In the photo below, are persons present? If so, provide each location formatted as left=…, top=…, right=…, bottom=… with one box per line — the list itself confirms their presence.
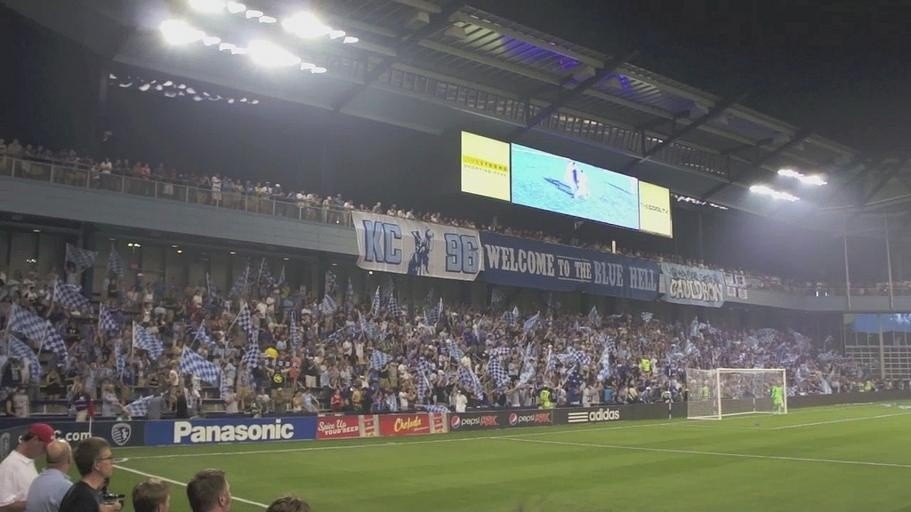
left=2, top=137, right=909, bottom=425
left=0, top=423, right=317, bottom=511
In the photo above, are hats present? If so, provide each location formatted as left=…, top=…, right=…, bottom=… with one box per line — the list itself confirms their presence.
left=30, top=423, right=55, bottom=448
